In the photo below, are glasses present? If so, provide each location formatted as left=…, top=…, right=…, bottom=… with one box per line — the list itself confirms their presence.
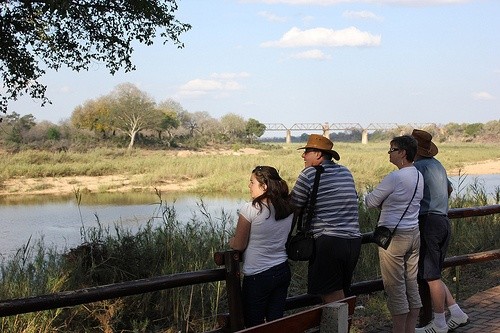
left=390, top=147, right=400, bottom=151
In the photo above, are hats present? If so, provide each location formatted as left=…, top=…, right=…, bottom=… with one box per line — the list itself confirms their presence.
left=296, top=134, right=340, bottom=161
left=411, top=129, right=438, bottom=157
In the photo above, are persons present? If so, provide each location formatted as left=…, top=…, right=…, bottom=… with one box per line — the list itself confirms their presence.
left=364, top=135, right=423, bottom=333
left=412, top=129, right=469, bottom=333
left=287, top=134, right=362, bottom=333
left=227, top=166, right=295, bottom=329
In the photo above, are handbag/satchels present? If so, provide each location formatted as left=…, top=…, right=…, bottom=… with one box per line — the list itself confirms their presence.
left=371, top=225, right=393, bottom=250
left=285, top=229, right=314, bottom=260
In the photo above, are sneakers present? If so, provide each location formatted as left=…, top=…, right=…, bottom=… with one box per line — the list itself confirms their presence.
left=415, top=320, right=448, bottom=333
left=447, top=313, right=470, bottom=330
